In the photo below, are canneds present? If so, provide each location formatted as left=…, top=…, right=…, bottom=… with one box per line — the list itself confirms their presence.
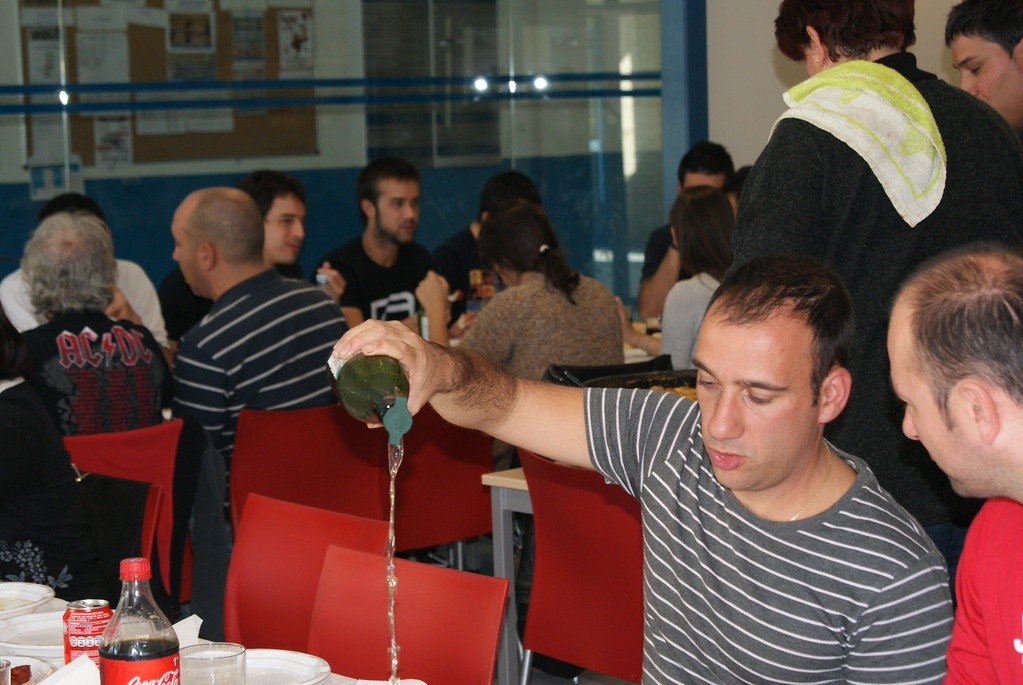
left=62, top=598, right=114, bottom=671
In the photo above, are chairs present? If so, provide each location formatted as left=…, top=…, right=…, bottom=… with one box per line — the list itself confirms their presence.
left=55, top=397, right=646, bottom=685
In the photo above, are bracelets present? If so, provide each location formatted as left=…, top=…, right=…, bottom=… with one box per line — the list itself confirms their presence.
left=670, top=242, right=678, bottom=250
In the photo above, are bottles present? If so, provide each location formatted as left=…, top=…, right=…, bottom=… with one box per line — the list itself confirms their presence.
left=98, top=557, right=181, bottom=685
left=417, top=309, right=430, bottom=342
left=326, top=349, right=416, bottom=448
left=316, top=274, right=340, bottom=305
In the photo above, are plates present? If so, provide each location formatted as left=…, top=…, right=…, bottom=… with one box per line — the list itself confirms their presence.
left=0, top=656, right=57, bottom=685
left=0, top=580, right=56, bottom=620
left=245, top=648, right=332, bottom=685
left=0, top=610, right=119, bottom=653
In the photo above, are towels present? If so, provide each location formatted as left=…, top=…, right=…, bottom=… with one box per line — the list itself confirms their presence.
left=764, top=58, right=949, bottom=228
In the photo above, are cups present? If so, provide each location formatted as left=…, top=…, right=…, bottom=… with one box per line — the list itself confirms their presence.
left=176, top=642, right=247, bottom=685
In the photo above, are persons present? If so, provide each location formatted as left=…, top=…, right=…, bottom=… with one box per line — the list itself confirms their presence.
left=173, top=186, right=351, bottom=549
left=727, top=0, right=1023, bottom=603
left=0, top=192, right=172, bottom=608
left=887, top=238, right=1023, bottom=685
left=317, top=159, right=432, bottom=329
left=432, top=169, right=543, bottom=337
left=726, top=166, right=754, bottom=209
left=614, top=185, right=734, bottom=369
left=416, top=196, right=624, bottom=573
left=332, top=256, right=954, bottom=685
left=637, top=140, right=735, bottom=335
left=159, top=169, right=346, bottom=350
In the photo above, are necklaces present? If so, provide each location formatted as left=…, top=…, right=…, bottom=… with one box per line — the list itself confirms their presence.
left=789, top=439, right=832, bottom=522
